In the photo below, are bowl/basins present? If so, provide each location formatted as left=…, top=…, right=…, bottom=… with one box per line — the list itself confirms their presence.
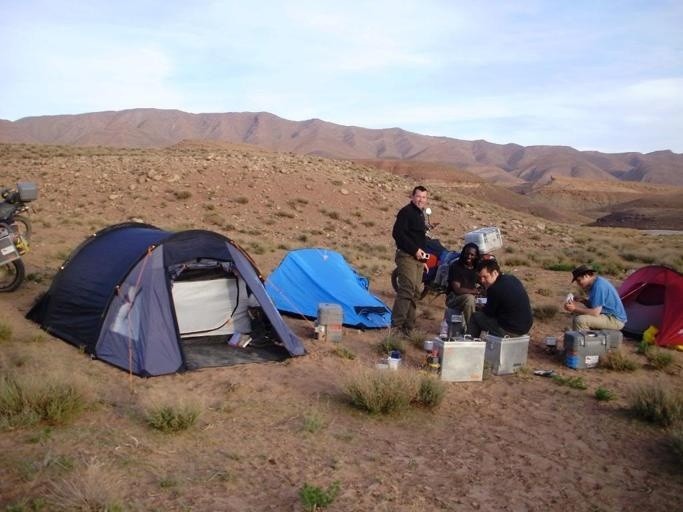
left=424, top=341, right=433, bottom=351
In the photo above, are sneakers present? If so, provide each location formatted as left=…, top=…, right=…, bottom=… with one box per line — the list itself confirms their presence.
left=399, top=326, right=410, bottom=339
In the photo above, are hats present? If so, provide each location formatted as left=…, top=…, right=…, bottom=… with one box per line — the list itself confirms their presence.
left=570, top=264, right=597, bottom=283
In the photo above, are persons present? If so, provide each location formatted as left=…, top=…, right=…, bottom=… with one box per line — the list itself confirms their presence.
left=389, top=185, right=430, bottom=338
left=445, top=244, right=485, bottom=330
left=564, top=265, right=628, bottom=333
left=467, top=259, right=534, bottom=340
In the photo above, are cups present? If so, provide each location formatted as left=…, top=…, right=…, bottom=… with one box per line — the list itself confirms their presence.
left=545, top=337, right=557, bottom=354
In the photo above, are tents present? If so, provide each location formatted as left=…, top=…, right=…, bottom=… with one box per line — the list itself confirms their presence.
left=263, top=244, right=392, bottom=332
left=25, top=221, right=310, bottom=380
left=616, top=263, right=682, bottom=350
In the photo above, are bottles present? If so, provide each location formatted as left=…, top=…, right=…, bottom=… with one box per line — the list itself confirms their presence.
left=440, top=319, right=448, bottom=338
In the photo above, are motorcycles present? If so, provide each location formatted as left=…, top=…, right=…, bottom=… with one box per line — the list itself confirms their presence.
left=391, top=207, right=504, bottom=300
left=0, top=183, right=37, bottom=292
